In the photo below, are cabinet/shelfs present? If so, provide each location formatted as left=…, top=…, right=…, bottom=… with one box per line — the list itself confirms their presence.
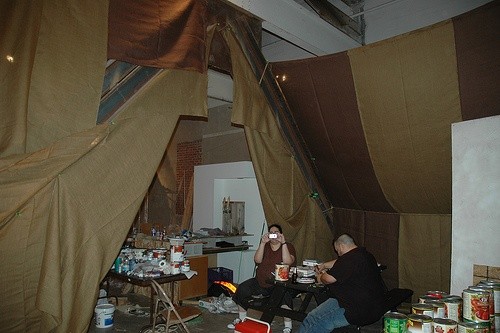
left=179, top=256, right=207, bottom=302
left=109, top=271, right=192, bottom=333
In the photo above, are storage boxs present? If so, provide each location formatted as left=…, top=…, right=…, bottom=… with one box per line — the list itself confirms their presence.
left=209, top=267, right=234, bottom=297
left=184, top=244, right=203, bottom=256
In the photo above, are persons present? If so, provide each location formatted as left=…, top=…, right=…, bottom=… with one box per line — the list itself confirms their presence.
left=227, top=223, right=296, bottom=333
left=296, top=233, right=386, bottom=333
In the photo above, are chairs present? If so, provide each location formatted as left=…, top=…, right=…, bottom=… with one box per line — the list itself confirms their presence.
left=152, top=280, right=201, bottom=333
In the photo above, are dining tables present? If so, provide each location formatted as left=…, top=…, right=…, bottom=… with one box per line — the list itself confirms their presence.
left=261, top=266, right=384, bottom=324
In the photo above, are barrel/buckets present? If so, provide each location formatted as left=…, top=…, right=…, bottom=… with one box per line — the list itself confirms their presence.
left=94, top=304, right=115, bottom=328
left=112, top=243, right=191, bottom=277
left=384, top=279, right=500, bottom=333
left=275, top=263, right=289, bottom=281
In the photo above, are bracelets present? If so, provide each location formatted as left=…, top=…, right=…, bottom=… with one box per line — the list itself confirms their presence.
left=282, top=242, right=288, bottom=245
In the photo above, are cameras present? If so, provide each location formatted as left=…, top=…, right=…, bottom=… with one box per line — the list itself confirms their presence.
left=268, top=234, right=277, bottom=238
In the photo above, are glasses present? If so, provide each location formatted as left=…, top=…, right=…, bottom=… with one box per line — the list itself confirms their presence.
left=268, top=231, right=279, bottom=234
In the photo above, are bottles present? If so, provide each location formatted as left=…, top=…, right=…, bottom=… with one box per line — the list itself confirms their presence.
left=292, top=267, right=298, bottom=283
left=152, top=224, right=166, bottom=240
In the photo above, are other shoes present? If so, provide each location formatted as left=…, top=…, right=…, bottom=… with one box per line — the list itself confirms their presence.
left=282, top=326, right=293, bottom=333
left=227, top=318, right=242, bottom=330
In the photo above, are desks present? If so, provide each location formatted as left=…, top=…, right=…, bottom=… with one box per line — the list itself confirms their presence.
left=202, top=245, right=254, bottom=283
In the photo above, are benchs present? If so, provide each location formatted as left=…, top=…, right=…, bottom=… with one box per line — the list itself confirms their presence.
left=248, top=288, right=412, bottom=330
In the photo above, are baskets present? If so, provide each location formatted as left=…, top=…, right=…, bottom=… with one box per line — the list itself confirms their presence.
left=207, top=266, right=234, bottom=298
